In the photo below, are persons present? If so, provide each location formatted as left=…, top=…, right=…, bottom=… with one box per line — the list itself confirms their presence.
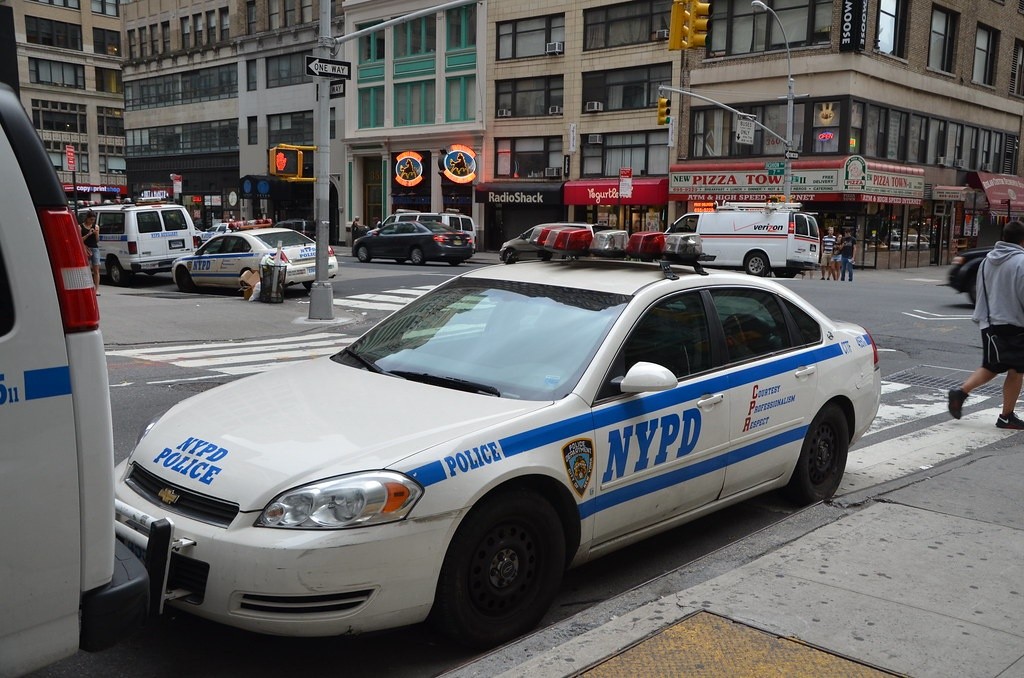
left=79, top=212, right=101, bottom=296
left=351, top=216, right=364, bottom=232
left=820, top=227, right=857, bottom=281
left=949, top=221, right=1024, bottom=429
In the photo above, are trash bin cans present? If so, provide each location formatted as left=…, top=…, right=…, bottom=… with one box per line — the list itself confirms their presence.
left=259, top=264, right=287, bottom=303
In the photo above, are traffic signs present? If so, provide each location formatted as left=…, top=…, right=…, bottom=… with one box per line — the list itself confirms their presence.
left=736, top=120, right=761, bottom=152
left=784, top=150, right=799, bottom=160
left=303, top=55, right=354, bottom=82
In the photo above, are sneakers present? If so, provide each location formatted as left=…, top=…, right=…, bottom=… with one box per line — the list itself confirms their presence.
left=948, top=388, right=969, bottom=419
left=997, top=410, right=1024, bottom=429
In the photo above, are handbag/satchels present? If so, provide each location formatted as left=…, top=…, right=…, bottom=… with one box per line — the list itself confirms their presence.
left=987, top=333, right=1024, bottom=363
left=84, top=244, right=92, bottom=257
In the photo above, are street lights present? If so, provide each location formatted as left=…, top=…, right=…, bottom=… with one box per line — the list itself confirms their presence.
left=751, top=0, right=795, bottom=202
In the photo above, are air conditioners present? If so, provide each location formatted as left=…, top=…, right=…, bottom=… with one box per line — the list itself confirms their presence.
left=588, top=134, right=603, bottom=144
left=655, top=29, right=669, bottom=39
left=953, top=159, right=965, bottom=168
left=546, top=42, right=563, bottom=55
left=982, top=163, right=991, bottom=172
left=937, top=157, right=947, bottom=166
left=498, top=108, right=508, bottom=117
left=545, top=167, right=561, bottom=177
left=549, top=105, right=562, bottom=114
left=587, top=101, right=603, bottom=111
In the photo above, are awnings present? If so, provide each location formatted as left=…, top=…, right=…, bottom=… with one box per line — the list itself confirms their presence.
left=564, top=178, right=668, bottom=204
left=966, top=171, right=1024, bottom=212
left=475, top=182, right=565, bottom=204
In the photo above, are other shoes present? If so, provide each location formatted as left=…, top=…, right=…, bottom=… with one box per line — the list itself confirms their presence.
left=96, top=292, right=101, bottom=296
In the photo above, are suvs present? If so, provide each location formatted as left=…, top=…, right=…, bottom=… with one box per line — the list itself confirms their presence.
left=0, top=84, right=157, bottom=677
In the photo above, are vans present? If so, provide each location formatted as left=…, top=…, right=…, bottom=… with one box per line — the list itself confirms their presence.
left=663, top=201, right=821, bottom=280
left=69, top=195, right=204, bottom=284
left=374, top=208, right=482, bottom=256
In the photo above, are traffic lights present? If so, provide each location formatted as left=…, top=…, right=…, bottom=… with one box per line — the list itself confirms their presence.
left=655, top=97, right=671, bottom=128
left=689, top=0, right=714, bottom=54
left=272, top=146, right=305, bottom=179
left=668, top=0, right=691, bottom=53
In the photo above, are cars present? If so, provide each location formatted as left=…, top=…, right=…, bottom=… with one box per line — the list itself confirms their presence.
left=170, top=220, right=338, bottom=293
left=890, top=233, right=947, bottom=251
left=944, top=246, right=999, bottom=304
left=499, top=224, right=613, bottom=265
left=117, top=223, right=885, bottom=658
left=350, top=222, right=472, bottom=264
